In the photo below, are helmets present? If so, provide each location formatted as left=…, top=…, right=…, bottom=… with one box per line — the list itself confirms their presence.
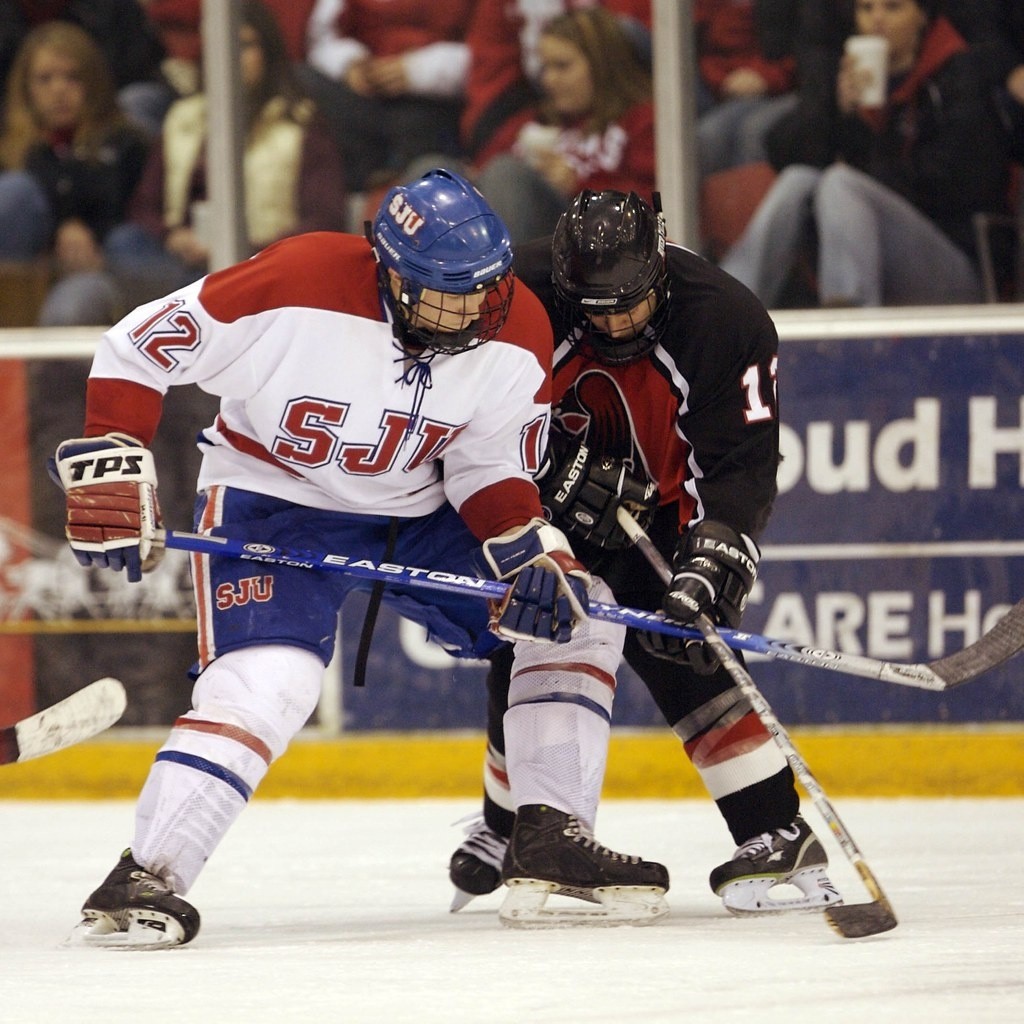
left=365, top=167, right=517, bottom=357
left=552, top=187, right=666, bottom=368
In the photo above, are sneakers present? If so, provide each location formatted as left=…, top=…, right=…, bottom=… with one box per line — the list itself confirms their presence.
left=501, top=803, right=671, bottom=926
left=447, top=791, right=518, bottom=913
left=709, top=813, right=844, bottom=913
left=71, top=848, right=201, bottom=951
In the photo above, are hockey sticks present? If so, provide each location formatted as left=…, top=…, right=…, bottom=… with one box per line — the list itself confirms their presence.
left=155, top=525, right=1024, bottom=693
left=614, top=505, right=900, bottom=940
left=1, top=678, right=130, bottom=766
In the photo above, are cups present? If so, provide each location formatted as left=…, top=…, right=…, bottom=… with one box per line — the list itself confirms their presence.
left=849, top=37, right=889, bottom=106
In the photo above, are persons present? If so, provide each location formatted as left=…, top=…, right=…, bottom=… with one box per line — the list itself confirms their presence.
left=57, top=165, right=671, bottom=953
left=447, top=192, right=844, bottom=910
left=717, top=1, right=1014, bottom=307
left=401, top=5, right=658, bottom=247
left=0, top=0, right=1023, bottom=324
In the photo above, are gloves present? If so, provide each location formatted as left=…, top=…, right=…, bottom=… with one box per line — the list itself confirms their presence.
left=638, top=521, right=762, bottom=678
left=50, top=432, right=166, bottom=582
left=545, top=438, right=659, bottom=552
left=471, top=516, right=591, bottom=647
